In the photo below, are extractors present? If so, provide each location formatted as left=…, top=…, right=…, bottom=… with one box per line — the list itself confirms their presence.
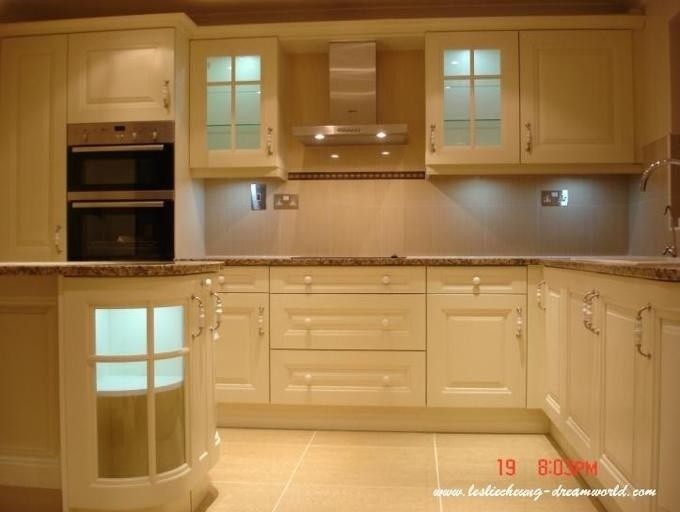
left=288, top=39, right=410, bottom=146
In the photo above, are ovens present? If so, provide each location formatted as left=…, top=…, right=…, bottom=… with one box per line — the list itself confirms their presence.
left=67, top=123, right=176, bottom=262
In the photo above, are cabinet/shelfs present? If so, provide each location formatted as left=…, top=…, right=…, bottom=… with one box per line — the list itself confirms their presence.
left=423, top=28, right=641, bottom=182
left=535, top=265, right=567, bottom=431
left=188, top=35, right=289, bottom=183
left=217, top=264, right=528, bottom=422
left=568, top=268, right=679, bottom=511
left=0, top=30, right=176, bottom=265
left=43, top=276, right=226, bottom=509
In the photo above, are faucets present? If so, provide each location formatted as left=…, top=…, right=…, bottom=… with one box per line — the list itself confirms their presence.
left=638, top=157, right=680, bottom=192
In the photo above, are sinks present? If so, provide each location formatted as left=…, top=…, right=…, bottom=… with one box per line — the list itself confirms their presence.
left=568, top=255, right=680, bottom=266
